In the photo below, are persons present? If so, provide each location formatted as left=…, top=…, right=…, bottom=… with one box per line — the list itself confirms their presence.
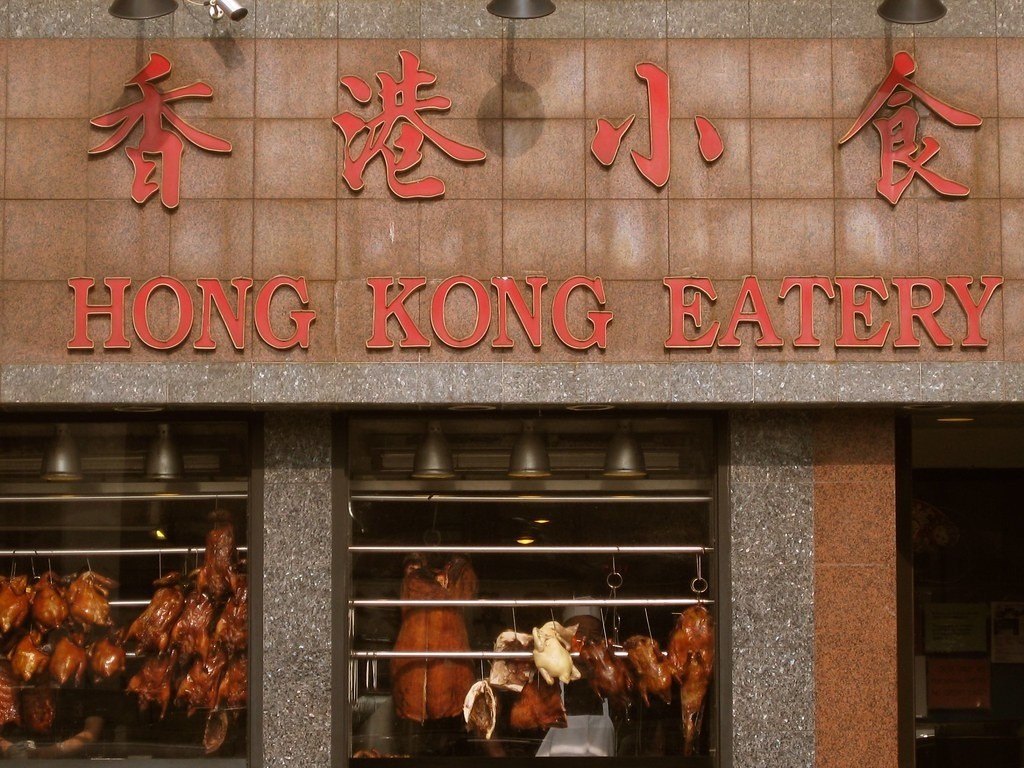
left=533, top=595, right=626, bottom=757
left=0, top=655, right=111, bottom=760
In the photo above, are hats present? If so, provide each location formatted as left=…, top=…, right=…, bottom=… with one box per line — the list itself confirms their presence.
left=563, top=596, right=601, bottom=622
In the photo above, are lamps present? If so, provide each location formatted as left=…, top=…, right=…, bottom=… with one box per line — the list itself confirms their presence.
left=108, top=0, right=179, bottom=20
left=877, top=0, right=947, bottom=25
left=603, top=416, right=648, bottom=477
left=142, top=422, right=184, bottom=481
left=506, top=418, right=551, bottom=477
left=41, top=422, right=86, bottom=479
left=412, top=418, right=457, bottom=479
left=486, top=0, right=556, bottom=18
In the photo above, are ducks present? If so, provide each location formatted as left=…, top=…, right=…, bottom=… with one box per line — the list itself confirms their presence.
left=1, top=509, right=249, bottom=754
left=389, top=553, right=716, bottom=756
